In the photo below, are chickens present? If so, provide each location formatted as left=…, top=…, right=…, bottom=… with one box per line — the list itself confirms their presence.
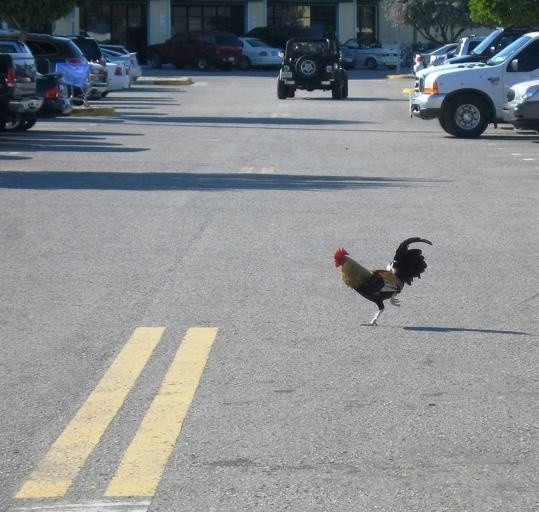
left=334, top=237, right=433, bottom=326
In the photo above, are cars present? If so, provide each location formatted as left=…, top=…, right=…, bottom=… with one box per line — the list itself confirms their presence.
left=409, top=26, right=538, bottom=138
left=145, top=30, right=243, bottom=71
left=237, top=36, right=282, bottom=71
left=1, top=29, right=144, bottom=133
left=342, top=38, right=399, bottom=70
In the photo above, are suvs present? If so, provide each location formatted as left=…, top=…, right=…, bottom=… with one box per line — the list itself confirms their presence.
left=276, top=36, right=349, bottom=101
